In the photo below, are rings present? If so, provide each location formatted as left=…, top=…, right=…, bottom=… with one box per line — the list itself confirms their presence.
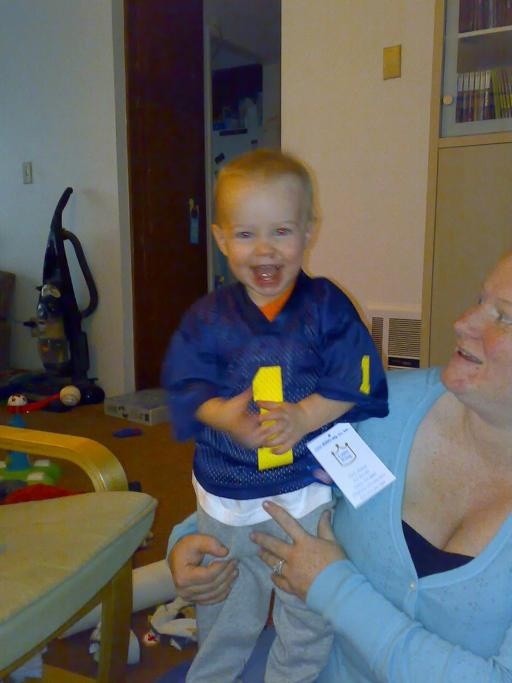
left=272, top=560, right=285, bottom=577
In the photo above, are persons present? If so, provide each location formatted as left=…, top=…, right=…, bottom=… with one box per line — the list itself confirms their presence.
left=164, top=251, right=512, bottom=683
left=158, top=150, right=389, bottom=683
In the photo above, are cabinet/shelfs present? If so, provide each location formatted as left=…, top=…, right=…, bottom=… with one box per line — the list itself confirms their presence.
left=419, top=3, right=512, bottom=368
